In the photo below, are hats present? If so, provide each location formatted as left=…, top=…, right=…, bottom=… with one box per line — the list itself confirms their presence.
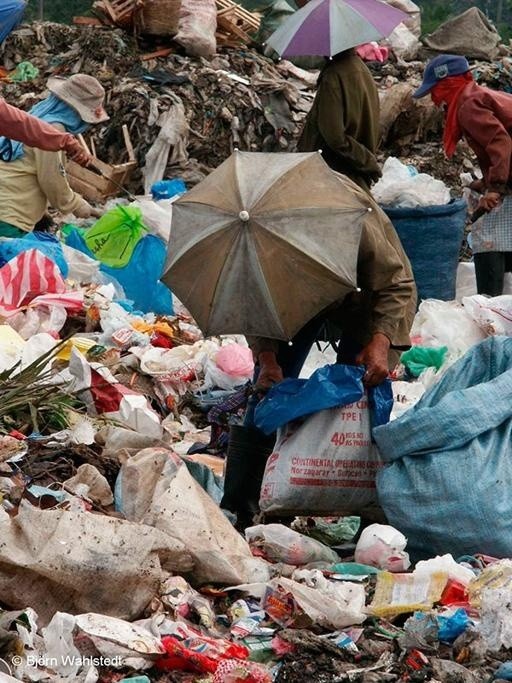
left=44, top=71, right=114, bottom=125
left=413, top=53, right=472, bottom=97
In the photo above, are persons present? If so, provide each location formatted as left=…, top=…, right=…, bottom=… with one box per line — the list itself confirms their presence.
left=296, top=48, right=382, bottom=202
left=0, top=74, right=110, bottom=238
left=219, top=170, right=417, bottom=533
left=412, top=54, right=512, bottom=296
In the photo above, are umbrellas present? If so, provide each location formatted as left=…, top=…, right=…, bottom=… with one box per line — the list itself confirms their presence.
left=262, top=0, right=416, bottom=60
left=157, top=148, right=372, bottom=346
